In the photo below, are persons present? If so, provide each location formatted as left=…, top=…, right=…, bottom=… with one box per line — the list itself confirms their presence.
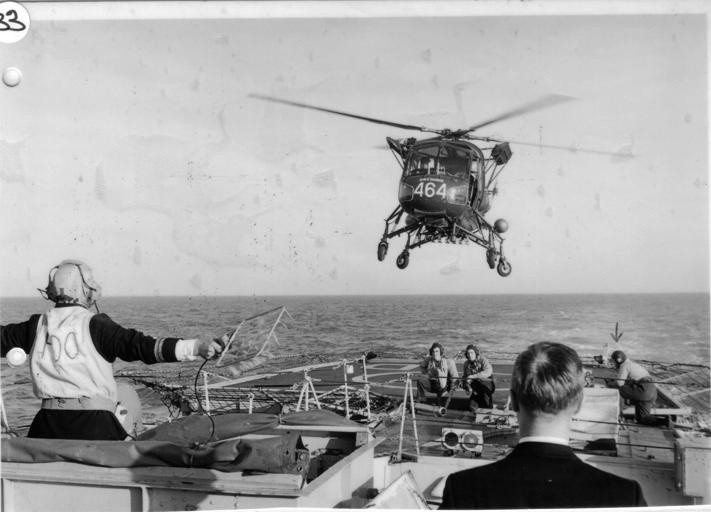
left=460, top=345, right=495, bottom=411
left=417, top=343, right=459, bottom=403
left=0, top=260, right=222, bottom=440
left=437, top=342, right=648, bottom=508
left=606, top=350, right=674, bottom=430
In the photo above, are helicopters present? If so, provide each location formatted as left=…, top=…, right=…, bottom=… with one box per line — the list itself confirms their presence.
left=248, top=92, right=635, bottom=277
